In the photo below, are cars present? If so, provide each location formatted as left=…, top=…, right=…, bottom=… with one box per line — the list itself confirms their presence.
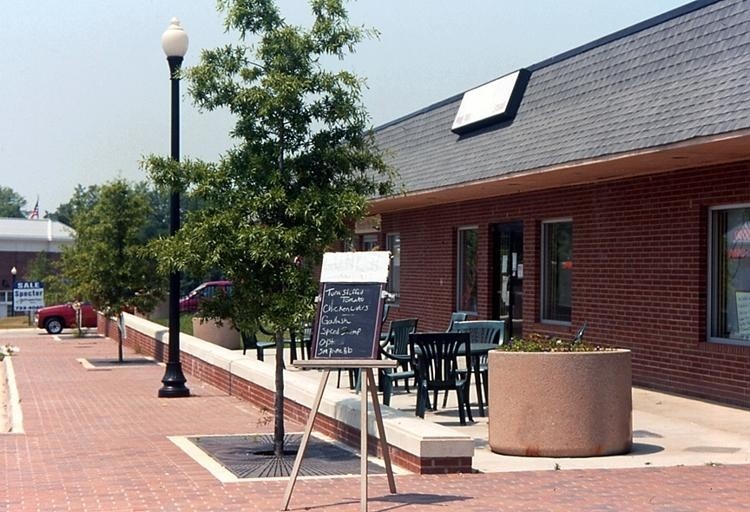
left=34, top=300, right=133, bottom=334
left=179, top=281, right=234, bottom=311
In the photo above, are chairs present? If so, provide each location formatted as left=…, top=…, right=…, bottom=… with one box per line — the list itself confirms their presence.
left=241, top=303, right=314, bottom=372
left=355, top=318, right=419, bottom=394
left=450, top=319, right=506, bottom=418
left=408, top=330, right=475, bottom=427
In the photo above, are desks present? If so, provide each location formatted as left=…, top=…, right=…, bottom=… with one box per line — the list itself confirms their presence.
left=379, top=331, right=473, bottom=344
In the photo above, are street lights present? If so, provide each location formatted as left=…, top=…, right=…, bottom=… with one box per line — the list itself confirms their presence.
left=157, top=15, right=191, bottom=397
left=10, top=267, right=17, bottom=316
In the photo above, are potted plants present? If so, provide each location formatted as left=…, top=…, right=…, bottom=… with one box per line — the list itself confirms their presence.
left=488, top=332, right=633, bottom=458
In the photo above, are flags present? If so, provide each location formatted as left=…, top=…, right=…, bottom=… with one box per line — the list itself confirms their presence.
left=29, top=201, right=39, bottom=219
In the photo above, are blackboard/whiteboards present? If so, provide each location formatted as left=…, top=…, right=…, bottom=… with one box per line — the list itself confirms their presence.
left=734, top=291, right=749, bottom=340
left=311, top=283, right=387, bottom=360
